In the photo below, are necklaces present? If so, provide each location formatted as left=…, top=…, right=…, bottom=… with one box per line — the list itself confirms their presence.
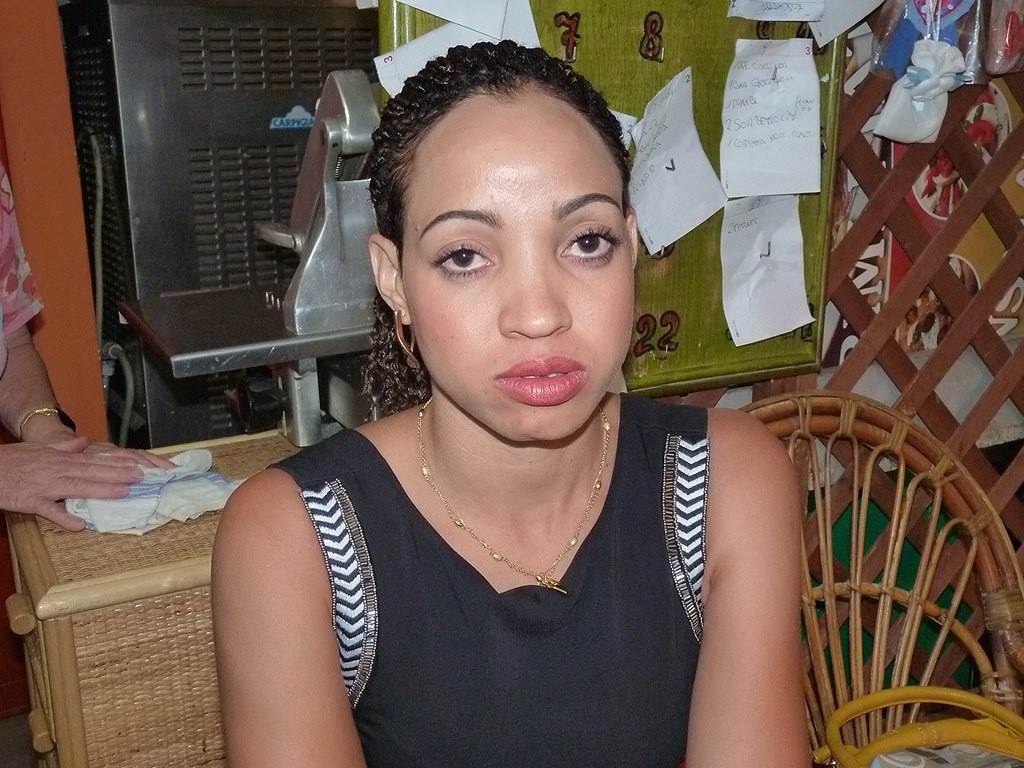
left=416, top=395, right=611, bottom=593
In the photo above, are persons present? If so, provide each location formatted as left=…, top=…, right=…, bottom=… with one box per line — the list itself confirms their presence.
left=0, top=160, right=176, bottom=531
left=210, top=38, right=812, bottom=768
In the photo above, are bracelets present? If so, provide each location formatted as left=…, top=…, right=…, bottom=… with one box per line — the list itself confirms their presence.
left=20, top=408, right=58, bottom=442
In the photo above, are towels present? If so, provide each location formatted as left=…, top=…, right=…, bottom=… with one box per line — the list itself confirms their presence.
left=65, top=448, right=249, bottom=537
